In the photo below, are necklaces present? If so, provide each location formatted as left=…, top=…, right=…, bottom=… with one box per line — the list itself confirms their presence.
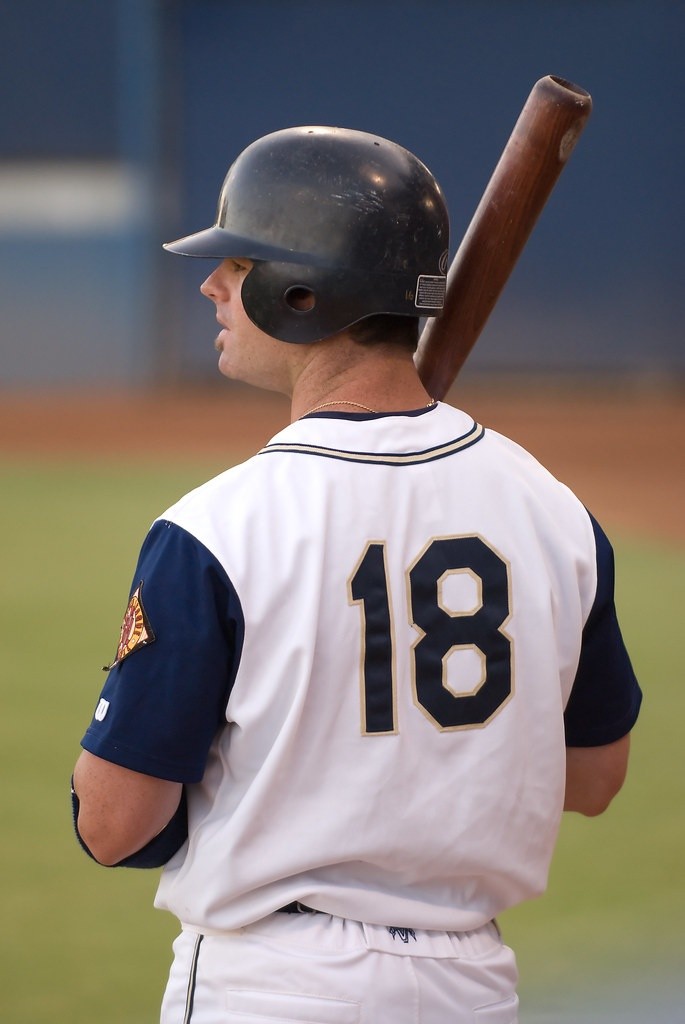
left=296, top=398, right=435, bottom=422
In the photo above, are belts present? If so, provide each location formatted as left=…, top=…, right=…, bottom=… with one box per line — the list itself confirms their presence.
left=276, top=901, right=325, bottom=914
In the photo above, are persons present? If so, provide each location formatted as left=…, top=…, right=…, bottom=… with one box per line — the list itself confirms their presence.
left=71, top=125, right=642, bottom=1024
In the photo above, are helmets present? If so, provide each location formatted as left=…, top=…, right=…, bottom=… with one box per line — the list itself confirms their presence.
left=162, top=126, right=450, bottom=344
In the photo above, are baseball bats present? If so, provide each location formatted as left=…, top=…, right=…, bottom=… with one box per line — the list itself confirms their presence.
left=412, top=72, right=596, bottom=404
